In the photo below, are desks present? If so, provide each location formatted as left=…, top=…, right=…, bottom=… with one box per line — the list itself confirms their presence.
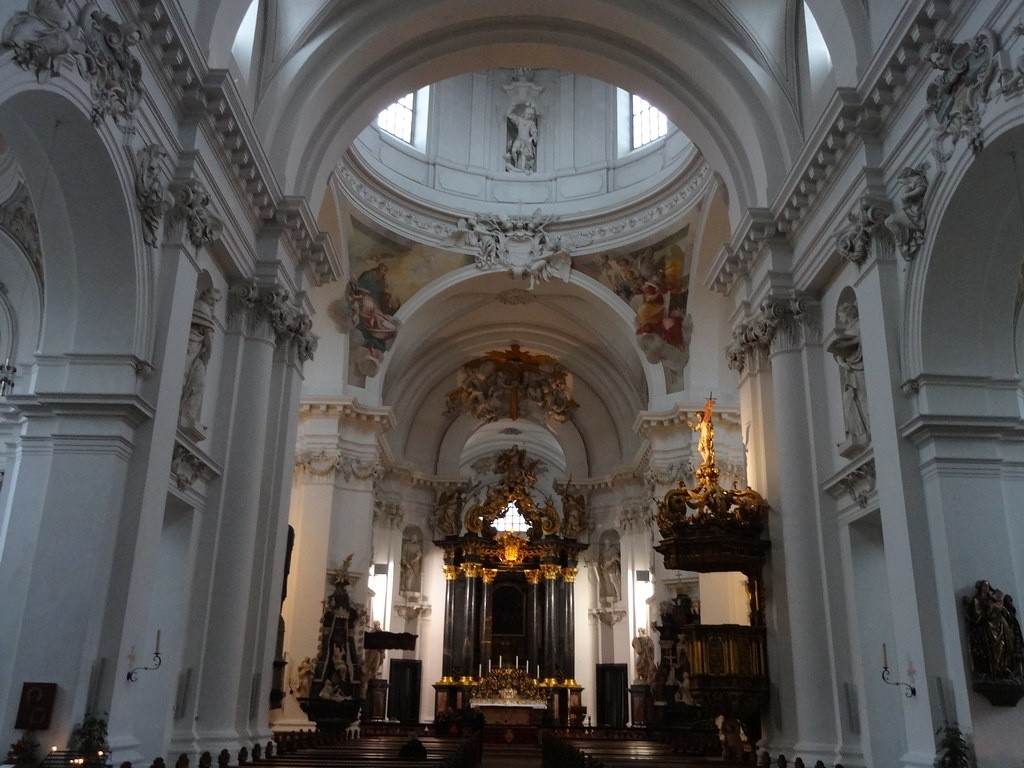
left=470, top=699, right=547, bottom=749
left=431, top=684, right=568, bottom=729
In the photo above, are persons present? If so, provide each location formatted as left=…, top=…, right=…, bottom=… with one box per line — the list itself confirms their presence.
left=832, top=41, right=969, bottom=261
left=833, top=300, right=870, bottom=447
left=503, top=73, right=548, bottom=172
left=599, top=247, right=687, bottom=353
left=178, top=287, right=226, bottom=432
left=600, top=538, right=622, bottom=603
left=666, top=633, right=696, bottom=707
left=294, top=622, right=385, bottom=702
left=966, top=579, right=1022, bottom=677
left=400, top=534, right=423, bottom=592
left=687, top=411, right=716, bottom=468
left=633, top=627, right=657, bottom=686
left=0, top=0, right=144, bottom=129
left=466, top=214, right=558, bottom=291
left=351, top=260, right=399, bottom=352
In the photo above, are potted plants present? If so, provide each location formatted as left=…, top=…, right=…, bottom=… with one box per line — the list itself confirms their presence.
left=80, top=715, right=108, bottom=764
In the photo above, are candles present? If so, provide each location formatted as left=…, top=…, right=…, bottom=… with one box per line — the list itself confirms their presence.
left=537, top=665, right=539, bottom=679
left=516, top=656, right=518, bottom=669
left=526, top=660, right=529, bottom=674
left=489, top=659, right=491, bottom=673
left=499, top=655, right=501, bottom=669
left=479, top=664, right=482, bottom=677
